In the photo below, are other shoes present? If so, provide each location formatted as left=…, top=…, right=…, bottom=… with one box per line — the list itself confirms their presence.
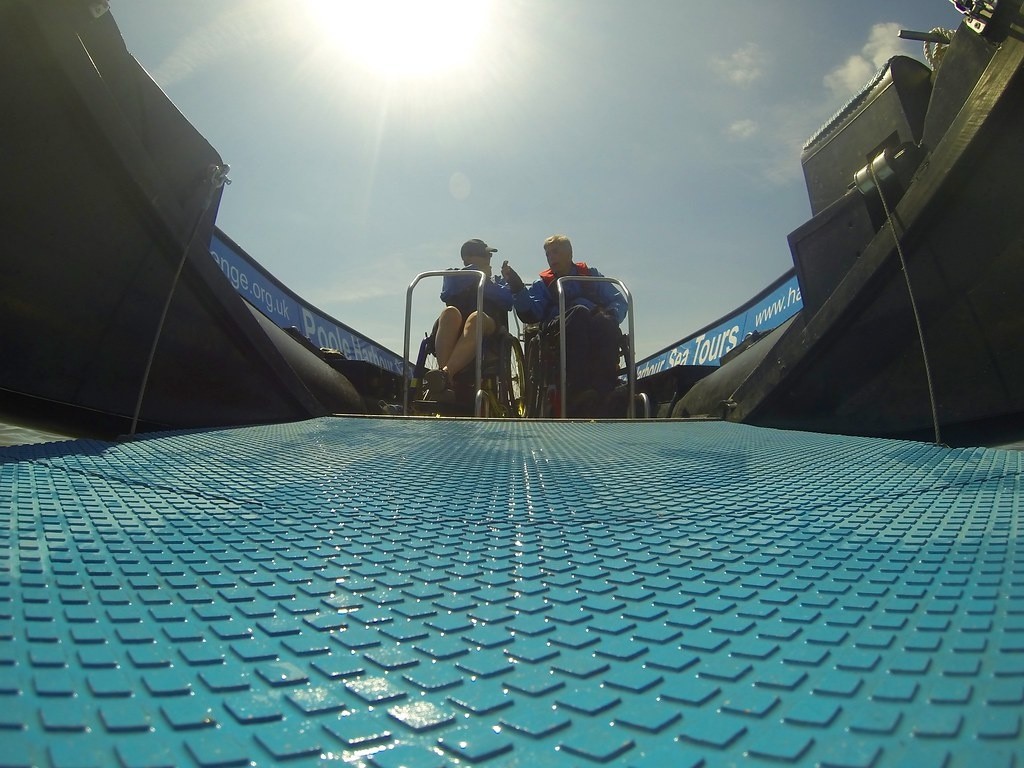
left=425, top=367, right=454, bottom=404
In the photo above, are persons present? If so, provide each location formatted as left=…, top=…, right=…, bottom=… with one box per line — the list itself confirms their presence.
left=501, top=235, right=628, bottom=412
left=425, top=239, right=513, bottom=394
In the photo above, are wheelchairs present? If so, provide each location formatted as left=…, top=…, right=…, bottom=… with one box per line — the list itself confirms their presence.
left=523, top=322, right=638, bottom=417
left=409, top=318, right=526, bottom=418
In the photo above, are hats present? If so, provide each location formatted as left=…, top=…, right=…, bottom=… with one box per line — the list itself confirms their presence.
left=461, top=240, right=499, bottom=258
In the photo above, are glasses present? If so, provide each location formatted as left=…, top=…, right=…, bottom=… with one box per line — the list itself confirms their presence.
left=470, top=253, right=494, bottom=258
